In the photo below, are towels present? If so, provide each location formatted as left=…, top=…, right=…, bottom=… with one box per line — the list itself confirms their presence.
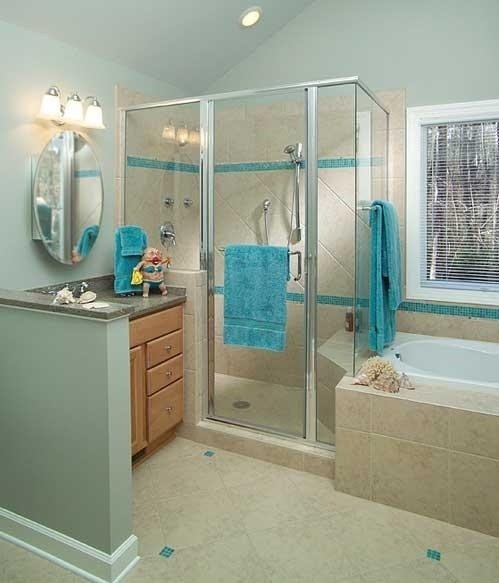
left=76, top=224, right=99, bottom=256
left=113, top=225, right=147, bottom=294
left=223, top=243, right=290, bottom=352
left=367, top=199, right=402, bottom=355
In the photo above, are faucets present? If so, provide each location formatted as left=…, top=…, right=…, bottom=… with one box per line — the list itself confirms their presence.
left=79, top=282, right=88, bottom=295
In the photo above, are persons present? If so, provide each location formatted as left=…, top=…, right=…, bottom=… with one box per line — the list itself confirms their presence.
left=133, top=247, right=172, bottom=297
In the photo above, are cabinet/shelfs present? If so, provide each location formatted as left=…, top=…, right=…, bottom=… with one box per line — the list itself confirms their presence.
left=129, top=304, right=184, bottom=470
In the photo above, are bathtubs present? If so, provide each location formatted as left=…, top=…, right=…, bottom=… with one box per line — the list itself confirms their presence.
left=384, top=335, right=498, bottom=388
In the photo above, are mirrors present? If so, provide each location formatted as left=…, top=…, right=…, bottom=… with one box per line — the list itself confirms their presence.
left=33, top=131, right=104, bottom=266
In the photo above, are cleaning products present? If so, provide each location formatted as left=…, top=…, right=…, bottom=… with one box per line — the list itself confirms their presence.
left=345, top=307, right=352, bottom=332
left=356, top=304, right=363, bottom=324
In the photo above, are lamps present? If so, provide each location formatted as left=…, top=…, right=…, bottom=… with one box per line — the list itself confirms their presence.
left=36, top=84, right=106, bottom=130
left=162, top=120, right=200, bottom=147
